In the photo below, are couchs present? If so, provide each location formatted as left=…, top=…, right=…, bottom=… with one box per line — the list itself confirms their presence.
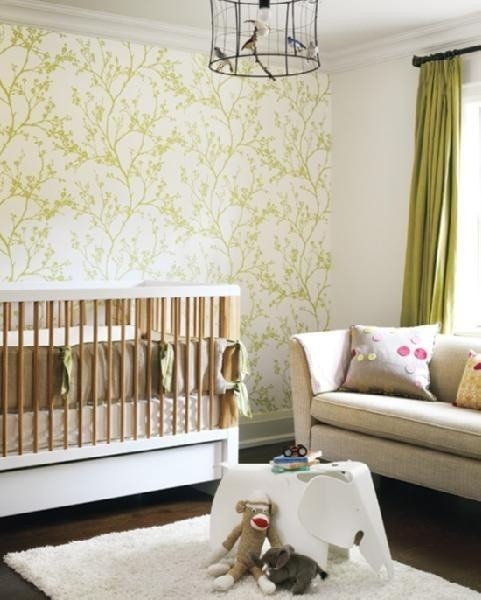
left=286, top=322, right=481, bottom=515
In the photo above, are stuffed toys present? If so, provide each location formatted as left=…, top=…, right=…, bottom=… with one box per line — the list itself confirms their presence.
left=260, top=546, right=327, bottom=595
left=206, top=493, right=282, bottom=593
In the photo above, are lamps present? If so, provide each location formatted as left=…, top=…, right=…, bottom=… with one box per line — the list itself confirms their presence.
left=209, top=0, right=322, bottom=81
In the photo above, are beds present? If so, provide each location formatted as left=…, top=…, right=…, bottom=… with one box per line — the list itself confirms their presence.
left=0, top=281, right=252, bottom=523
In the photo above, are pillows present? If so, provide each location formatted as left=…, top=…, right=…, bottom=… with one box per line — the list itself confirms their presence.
left=335, top=325, right=440, bottom=402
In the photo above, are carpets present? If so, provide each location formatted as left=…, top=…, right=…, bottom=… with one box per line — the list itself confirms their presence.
left=3, top=508, right=481, bottom=600
